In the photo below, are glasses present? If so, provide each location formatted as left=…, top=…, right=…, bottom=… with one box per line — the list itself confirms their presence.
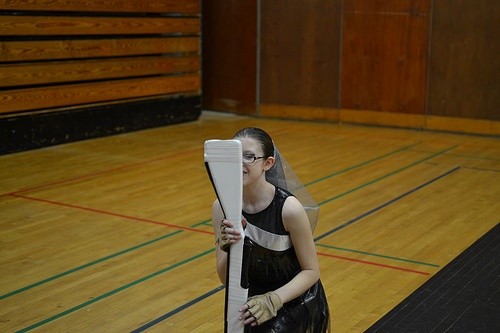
left=242, top=154, right=266, bottom=163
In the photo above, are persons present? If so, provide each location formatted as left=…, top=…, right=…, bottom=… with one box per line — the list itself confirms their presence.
left=211, top=126, right=331, bottom=333
